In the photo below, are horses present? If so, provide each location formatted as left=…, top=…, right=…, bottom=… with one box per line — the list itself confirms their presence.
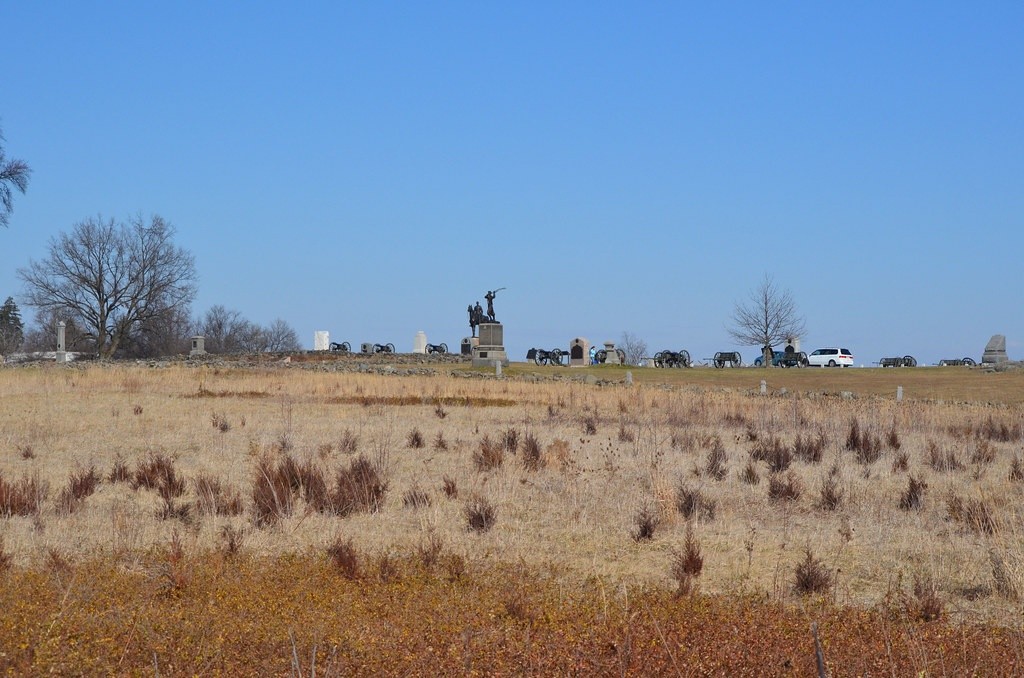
left=467, top=305, right=490, bottom=338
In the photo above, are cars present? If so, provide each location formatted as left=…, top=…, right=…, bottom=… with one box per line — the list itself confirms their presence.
left=753, top=351, right=798, bottom=367
left=803, top=348, right=854, bottom=367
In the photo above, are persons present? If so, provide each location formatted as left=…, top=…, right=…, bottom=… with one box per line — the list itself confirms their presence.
left=597, top=350, right=601, bottom=364
left=590, top=346, right=595, bottom=364
left=485, top=291, right=495, bottom=306
left=474, top=301, right=483, bottom=323
